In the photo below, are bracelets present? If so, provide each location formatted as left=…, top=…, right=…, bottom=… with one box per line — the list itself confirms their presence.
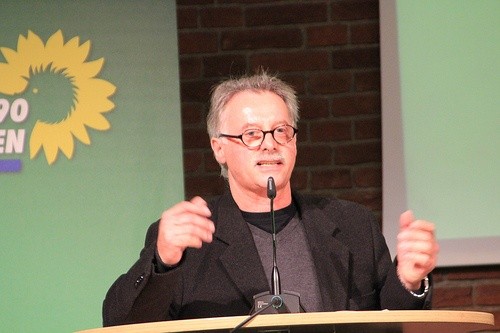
left=406, top=277, right=430, bottom=298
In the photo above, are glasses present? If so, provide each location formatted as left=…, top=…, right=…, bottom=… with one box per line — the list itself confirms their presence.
left=218, top=124, right=300, bottom=147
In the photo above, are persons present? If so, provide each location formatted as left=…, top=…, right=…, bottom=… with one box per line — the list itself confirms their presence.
left=102, top=70, right=439, bottom=328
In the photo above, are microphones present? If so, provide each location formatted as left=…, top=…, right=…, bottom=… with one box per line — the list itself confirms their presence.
left=253, top=177, right=300, bottom=314
